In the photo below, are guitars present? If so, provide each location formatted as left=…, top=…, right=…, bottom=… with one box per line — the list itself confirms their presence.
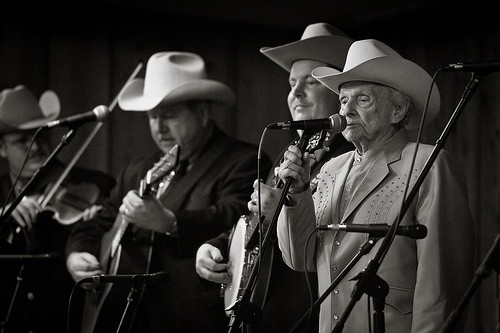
left=224, top=129, right=330, bottom=317
left=79, top=142, right=183, bottom=333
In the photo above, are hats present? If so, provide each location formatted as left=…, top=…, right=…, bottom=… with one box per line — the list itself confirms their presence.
left=117, top=51, right=236, bottom=119
left=311, top=39, right=440, bottom=131
left=260, top=22, right=354, bottom=71
left=0, top=86, right=58, bottom=133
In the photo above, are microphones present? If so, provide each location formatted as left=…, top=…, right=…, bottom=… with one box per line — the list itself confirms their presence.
left=88, top=271, right=171, bottom=283
left=448, top=62, right=500, bottom=74
left=266, top=113, right=347, bottom=133
left=318, top=224, right=428, bottom=239
left=43, top=104, right=110, bottom=128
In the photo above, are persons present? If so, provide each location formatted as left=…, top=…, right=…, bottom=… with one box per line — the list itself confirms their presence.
left=65, top=50, right=272, bottom=333
left=0, top=86, right=116, bottom=333
left=275, top=39, right=475, bottom=333
left=195, top=23, right=358, bottom=332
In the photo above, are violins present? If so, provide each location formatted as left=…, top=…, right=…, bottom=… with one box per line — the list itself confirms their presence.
left=16, top=167, right=114, bottom=232
